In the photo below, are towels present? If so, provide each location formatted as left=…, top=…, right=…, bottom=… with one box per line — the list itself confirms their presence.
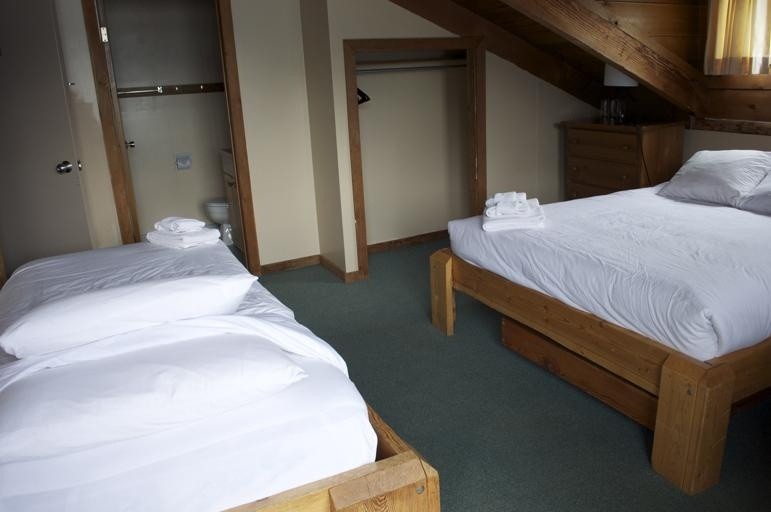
left=144, top=214, right=221, bottom=253
left=480, top=183, right=545, bottom=235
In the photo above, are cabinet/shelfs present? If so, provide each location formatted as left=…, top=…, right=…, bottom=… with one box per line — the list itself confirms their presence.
left=550, top=111, right=684, bottom=204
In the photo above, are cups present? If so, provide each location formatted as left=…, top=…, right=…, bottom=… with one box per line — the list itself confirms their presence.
left=599, top=91, right=625, bottom=124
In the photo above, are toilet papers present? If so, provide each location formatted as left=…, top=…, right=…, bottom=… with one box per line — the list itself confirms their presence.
left=176, top=157, right=190, bottom=169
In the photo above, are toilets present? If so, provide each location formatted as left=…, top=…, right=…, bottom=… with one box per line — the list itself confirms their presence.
left=203, top=201, right=229, bottom=225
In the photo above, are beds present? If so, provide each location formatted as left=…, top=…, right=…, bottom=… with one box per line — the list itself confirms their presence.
left=427, top=116, right=771, bottom=498
left=1, top=242, right=440, bottom=510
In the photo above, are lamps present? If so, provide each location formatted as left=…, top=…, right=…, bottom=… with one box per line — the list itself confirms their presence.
left=603, top=57, right=638, bottom=124
